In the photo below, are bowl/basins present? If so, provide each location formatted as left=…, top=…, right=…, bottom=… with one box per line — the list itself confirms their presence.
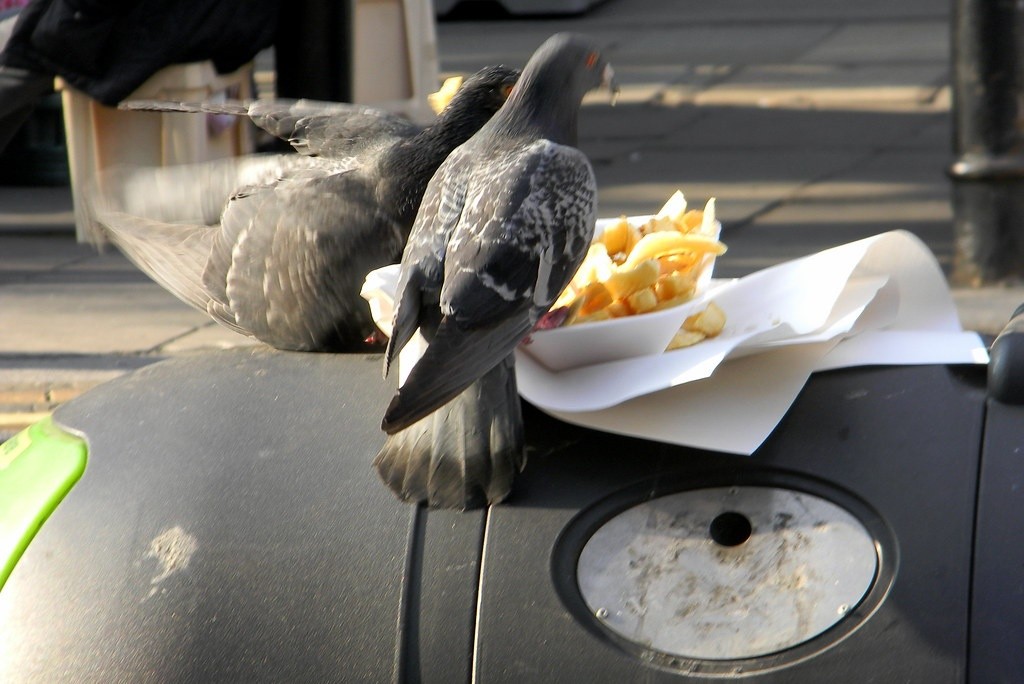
left=518, top=212, right=723, bottom=376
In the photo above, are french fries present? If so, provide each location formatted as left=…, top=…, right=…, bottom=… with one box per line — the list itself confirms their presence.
left=559, top=202, right=725, bottom=348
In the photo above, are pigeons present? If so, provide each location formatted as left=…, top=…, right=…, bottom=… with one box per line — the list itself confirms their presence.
left=95, top=65, right=522, bottom=354
left=370, top=32, right=622, bottom=516
